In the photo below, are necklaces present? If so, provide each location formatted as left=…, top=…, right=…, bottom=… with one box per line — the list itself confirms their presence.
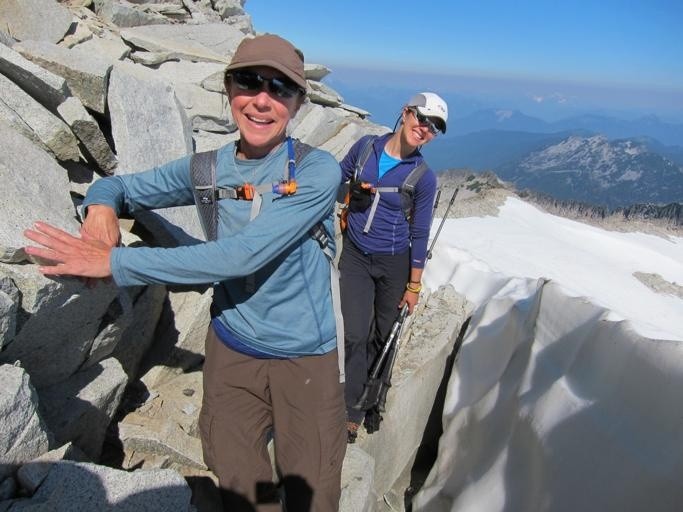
left=231, top=138, right=281, bottom=184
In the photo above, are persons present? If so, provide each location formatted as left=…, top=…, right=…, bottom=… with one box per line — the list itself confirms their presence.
left=339, top=91, right=449, bottom=445
left=22, top=32, right=348, bottom=511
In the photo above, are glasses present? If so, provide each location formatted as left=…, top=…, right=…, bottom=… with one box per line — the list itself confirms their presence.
left=408, top=107, right=440, bottom=135
left=231, top=71, right=299, bottom=97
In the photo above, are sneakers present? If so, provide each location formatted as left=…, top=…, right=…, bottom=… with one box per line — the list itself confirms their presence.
left=347, top=407, right=383, bottom=443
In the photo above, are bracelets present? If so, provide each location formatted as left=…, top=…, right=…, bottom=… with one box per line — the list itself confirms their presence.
left=409, top=280, right=421, bottom=286
left=406, top=282, right=421, bottom=293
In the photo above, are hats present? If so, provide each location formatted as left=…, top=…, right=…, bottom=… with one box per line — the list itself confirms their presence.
left=225, top=34, right=307, bottom=89
left=404, top=92, right=449, bottom=134
